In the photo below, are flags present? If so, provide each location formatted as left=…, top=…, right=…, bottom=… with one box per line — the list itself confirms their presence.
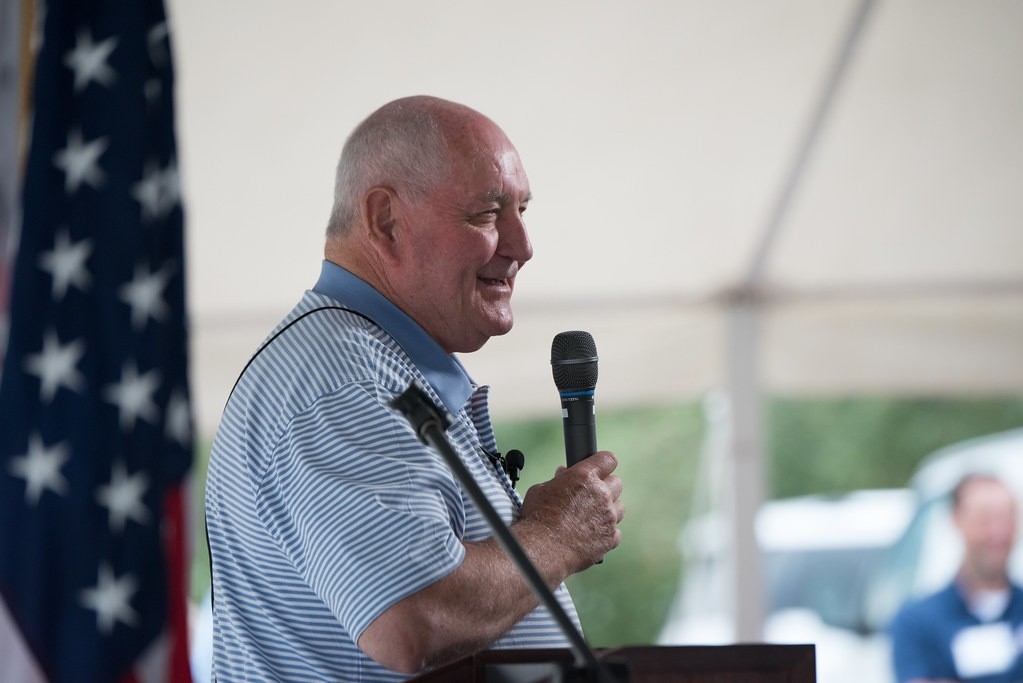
left=0, top=0, right=204, bottom=683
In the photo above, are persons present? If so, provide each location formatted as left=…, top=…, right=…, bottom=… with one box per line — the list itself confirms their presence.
left=890, top=472, right=1023, bottom=683
left=204, top=95, right=624, bottom=683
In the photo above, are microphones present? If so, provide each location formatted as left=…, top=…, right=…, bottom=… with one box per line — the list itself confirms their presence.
left=550, top=331, right=603, bottom=564
left=506, top=449, right=524, bottom=490
